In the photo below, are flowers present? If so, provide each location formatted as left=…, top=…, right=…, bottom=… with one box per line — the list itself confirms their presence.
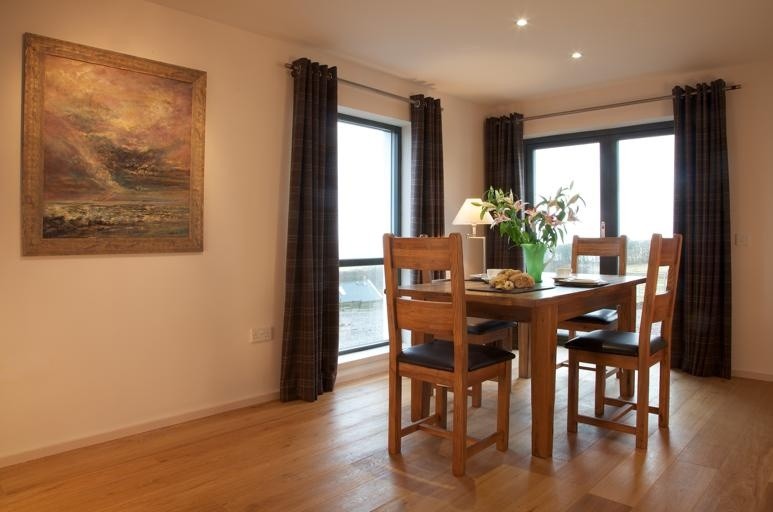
left=471, top=181, right=587, bottom=253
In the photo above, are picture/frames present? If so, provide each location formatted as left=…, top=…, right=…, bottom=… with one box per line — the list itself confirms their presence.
left=20, top=32, right=207, bottom=257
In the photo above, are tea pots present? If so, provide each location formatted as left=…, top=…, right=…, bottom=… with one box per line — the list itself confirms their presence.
left=520, top=243, right=555, bottom=283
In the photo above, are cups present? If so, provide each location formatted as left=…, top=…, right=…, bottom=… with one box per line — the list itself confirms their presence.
left=556, top=263, right=571, bottom=277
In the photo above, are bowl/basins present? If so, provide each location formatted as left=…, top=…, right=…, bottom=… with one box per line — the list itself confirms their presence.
left=486, top=269, right=504, bottom=279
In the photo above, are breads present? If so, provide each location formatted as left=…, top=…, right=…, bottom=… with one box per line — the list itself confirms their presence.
left=488, top=269, right=535, bottom=291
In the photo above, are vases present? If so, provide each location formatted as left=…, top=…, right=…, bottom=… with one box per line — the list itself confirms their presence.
left=520, top=243, right=547, bottom=283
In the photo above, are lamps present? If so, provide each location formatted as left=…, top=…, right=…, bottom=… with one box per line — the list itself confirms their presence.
left=452, top=198, right=496, bottom=238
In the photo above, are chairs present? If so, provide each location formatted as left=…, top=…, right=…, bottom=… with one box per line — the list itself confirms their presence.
left=565, top=233, right=683, bottom=449
left=419, top=235, right=516, bottom=408
left=383, top=232, right=516, bottom=477
left=558, top=235, right=627, bottom=397
